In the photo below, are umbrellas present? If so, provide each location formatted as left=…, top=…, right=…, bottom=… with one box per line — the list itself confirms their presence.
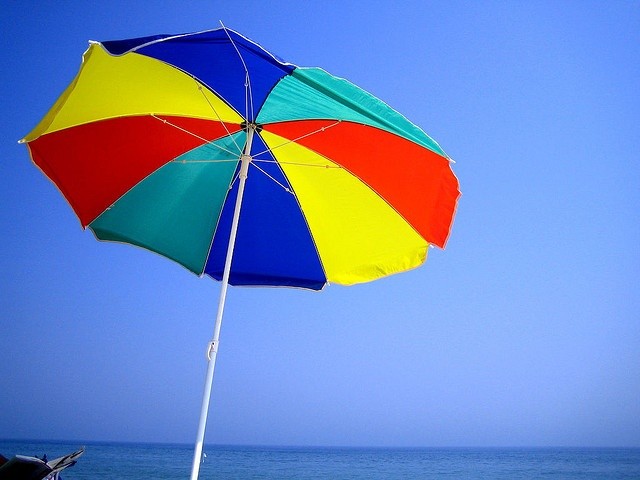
left=15, top=20, right=463, bottom=480
